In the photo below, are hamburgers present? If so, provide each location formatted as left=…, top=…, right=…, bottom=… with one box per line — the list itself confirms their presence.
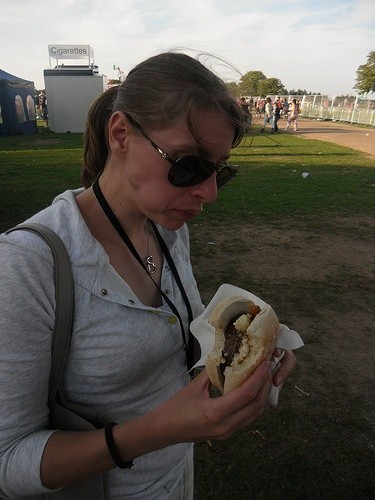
left=206, top=294, right=280, bottom=396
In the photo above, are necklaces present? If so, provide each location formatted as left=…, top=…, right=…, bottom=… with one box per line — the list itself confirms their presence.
left=90, top=187, right=158, bottom=275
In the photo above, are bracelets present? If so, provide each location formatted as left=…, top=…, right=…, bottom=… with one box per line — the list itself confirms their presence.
left=104, top=421, right=134, bottom=469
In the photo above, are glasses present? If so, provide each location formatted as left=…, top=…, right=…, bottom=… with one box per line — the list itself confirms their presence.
left=122, top=110, right=240, bottom=188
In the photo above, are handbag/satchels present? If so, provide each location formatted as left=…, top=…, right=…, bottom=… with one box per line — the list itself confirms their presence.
left=3, top=221, right=110, bottom=500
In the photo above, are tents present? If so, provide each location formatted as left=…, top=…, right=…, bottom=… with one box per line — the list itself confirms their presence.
left=0, top=69, right=38, bottom=136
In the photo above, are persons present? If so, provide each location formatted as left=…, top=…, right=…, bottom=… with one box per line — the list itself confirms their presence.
left=39, top=90, right=49, bottom=128
left=237, top=94, right=300, bottom=133
left=0, top=52, right=299, bottom=499
